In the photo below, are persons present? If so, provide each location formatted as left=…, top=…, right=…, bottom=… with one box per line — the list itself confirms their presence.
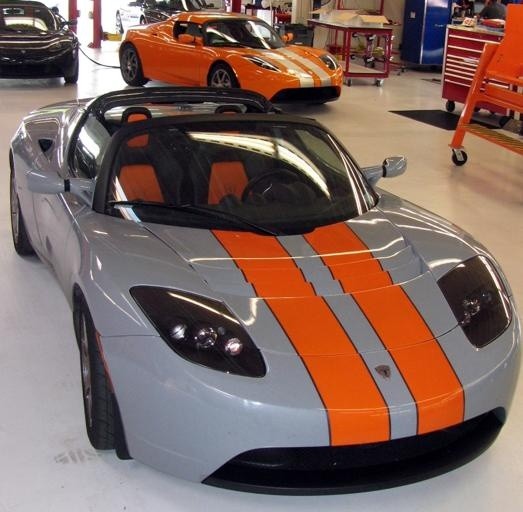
left=451, top=0, right=472, bottom=23
left=478, top=0, right=506, bottom=19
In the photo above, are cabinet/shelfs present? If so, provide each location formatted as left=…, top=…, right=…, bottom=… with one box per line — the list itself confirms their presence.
left=402, top=0, right=452, bottom=66
left=441, top=23, right=517, bottom=127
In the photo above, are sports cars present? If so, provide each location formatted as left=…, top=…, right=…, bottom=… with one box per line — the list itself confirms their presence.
left=8, top=87, right=521, bottom=496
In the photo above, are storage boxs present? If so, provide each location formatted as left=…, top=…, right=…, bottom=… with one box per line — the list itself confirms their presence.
left=308, top=8, right=369, bottom=23
left=336, top=11, right=389, bottom=29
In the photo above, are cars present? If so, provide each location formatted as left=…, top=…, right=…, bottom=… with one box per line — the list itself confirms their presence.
left=119, top=11, right=344, bottom=104
left=0, top=0, right=78, bottom=83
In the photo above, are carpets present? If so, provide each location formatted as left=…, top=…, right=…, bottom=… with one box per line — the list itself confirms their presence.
left=387, top=109, right=501, bottom=131
left=421, top=77, right=442, bottom=84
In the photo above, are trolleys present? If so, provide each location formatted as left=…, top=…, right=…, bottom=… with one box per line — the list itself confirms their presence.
left=307, top=19, right=402, bottom=87
left=440, top=24, right=509, bottom=115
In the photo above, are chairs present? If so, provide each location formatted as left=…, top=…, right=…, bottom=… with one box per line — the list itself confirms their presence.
left=194, top=105, right=254, bottom=208
left=115, top=105, right=168, bottom=205
